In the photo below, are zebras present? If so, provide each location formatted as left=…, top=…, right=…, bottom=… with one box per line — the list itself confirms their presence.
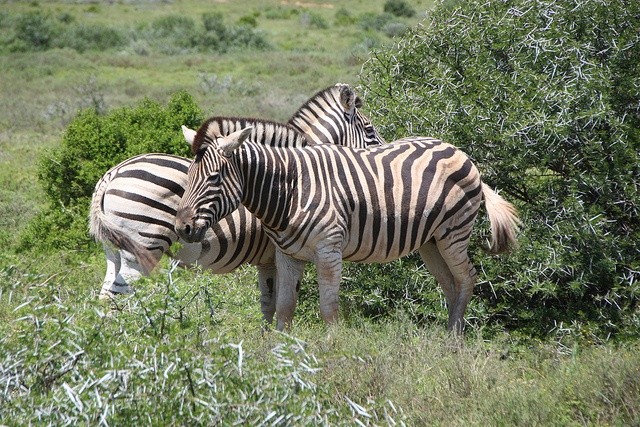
left=171, top=114, right=522, bottom=340
left=84, top=81, right=389, bottom=334
